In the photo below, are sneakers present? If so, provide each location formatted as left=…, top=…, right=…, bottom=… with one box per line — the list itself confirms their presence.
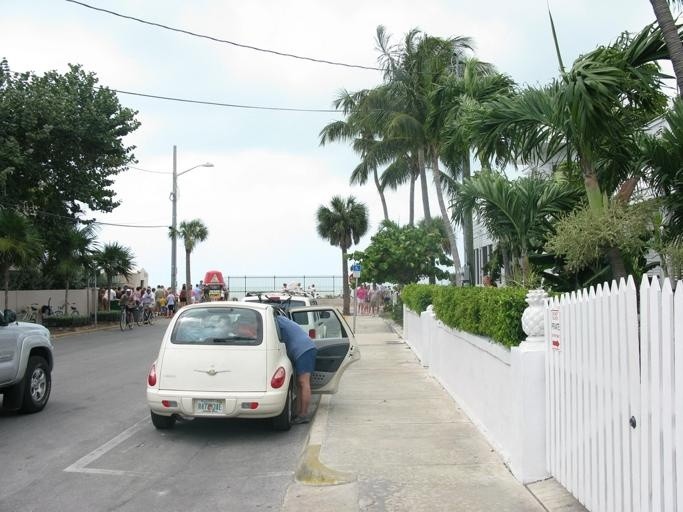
left=292, top=414, right=310, bottom=424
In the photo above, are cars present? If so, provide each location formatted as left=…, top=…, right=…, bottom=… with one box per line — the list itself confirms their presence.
left=147, top=291, right=361, bottom=431
left=0, top=309, right=54, bottom=413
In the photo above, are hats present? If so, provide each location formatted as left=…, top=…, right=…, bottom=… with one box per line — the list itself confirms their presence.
left=125, top=289, right=131, bottom=292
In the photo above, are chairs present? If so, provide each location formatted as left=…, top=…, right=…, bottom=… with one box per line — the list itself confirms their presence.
left=177, top=313, right=258, bottom=341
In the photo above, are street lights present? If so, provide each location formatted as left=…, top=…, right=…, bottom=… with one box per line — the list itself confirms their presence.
left=171, top=144, right=215, bottom=289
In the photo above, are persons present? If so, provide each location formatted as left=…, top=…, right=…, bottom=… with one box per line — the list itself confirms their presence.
left=354, top=283, right=398, bottom=317
left=100, top=280, right=204, bottom=327
left=275, top=313, right=316, bottom=425
left=281, top=282, right=316, bottom=296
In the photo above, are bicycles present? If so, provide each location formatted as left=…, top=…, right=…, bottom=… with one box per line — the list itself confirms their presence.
left=119, top=304, right=155, bottom=331
left=55, top=302, right=79, bottom=317
